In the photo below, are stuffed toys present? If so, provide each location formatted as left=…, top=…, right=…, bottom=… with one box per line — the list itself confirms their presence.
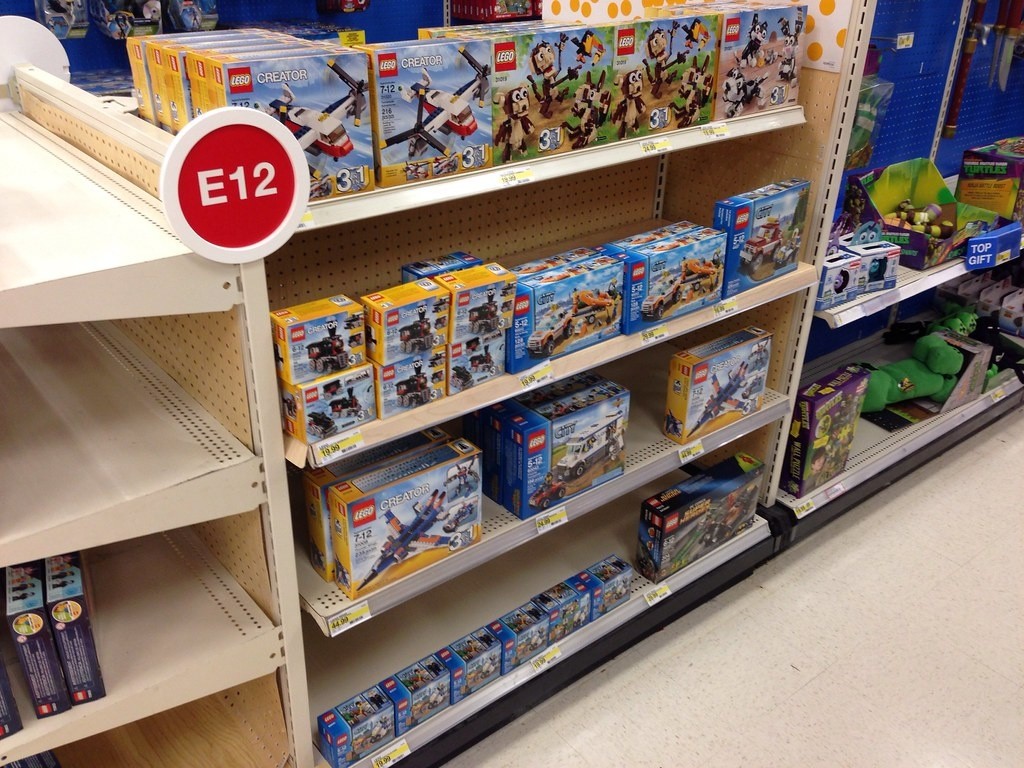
left=861, top=312, right=998, bottom=412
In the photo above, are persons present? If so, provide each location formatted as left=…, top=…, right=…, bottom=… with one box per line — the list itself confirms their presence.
left=443, top=455, right=480, bottom=500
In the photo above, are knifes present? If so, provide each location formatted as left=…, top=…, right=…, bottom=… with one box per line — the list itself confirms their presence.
left=988, top=0, right=1023, bottom=91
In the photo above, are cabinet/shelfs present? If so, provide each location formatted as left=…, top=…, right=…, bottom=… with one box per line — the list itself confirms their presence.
left=775, top=235, right=1024, bottom=520
left=0, top=112, right=297, bottom=768
left=285, top=101, right=819, bottom=768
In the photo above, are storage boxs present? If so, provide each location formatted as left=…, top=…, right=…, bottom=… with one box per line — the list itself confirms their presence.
left=0, top=2, right=1024, bottom=767
